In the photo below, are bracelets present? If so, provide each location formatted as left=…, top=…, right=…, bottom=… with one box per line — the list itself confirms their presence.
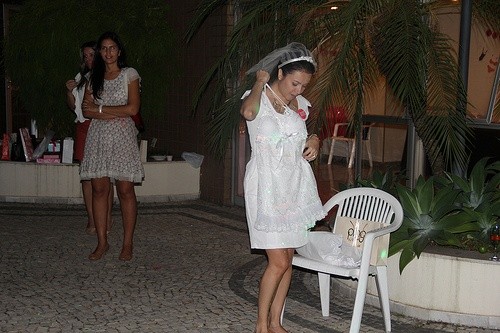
left=99, top=104, right=104, bottom=114
left=308, top=134, right=320, bottom=140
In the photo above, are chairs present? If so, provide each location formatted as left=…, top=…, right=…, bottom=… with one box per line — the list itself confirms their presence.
left=280, top=187, right=404, bottom=333
left=327, top=114, right=376, bottom=169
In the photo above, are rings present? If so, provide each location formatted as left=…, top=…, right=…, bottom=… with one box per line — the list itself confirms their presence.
left=313, top=155, right=317, bottom=158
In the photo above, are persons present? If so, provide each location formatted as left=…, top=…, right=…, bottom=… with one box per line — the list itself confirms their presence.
left=240, top=42, right=328, bottom=333
left=81, top=32, right=146, bottom=261
left=66, top=41, right=113, bottom=235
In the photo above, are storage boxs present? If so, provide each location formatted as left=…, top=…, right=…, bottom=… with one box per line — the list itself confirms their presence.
left=62, top=137, right=74, bottom=164
